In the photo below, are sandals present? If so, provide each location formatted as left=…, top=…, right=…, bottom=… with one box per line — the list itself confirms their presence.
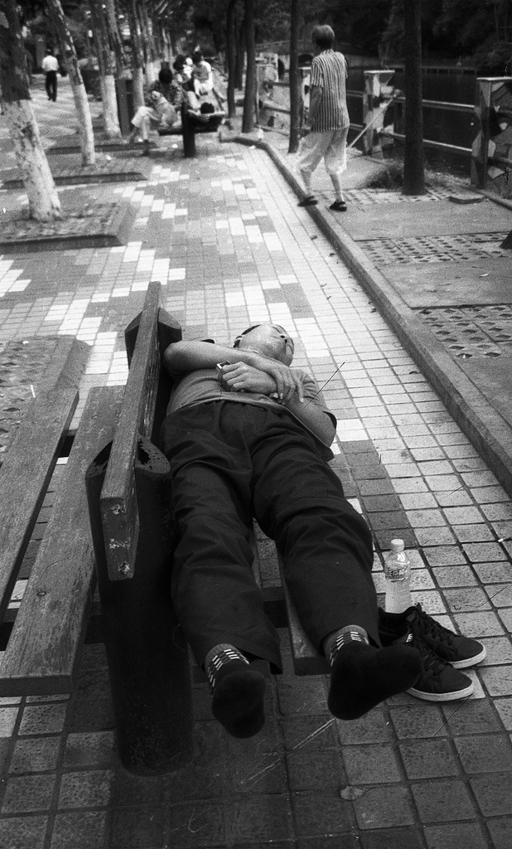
left=298, top=195, right=318, bottom=206
left=330, top=200, right=347, bottom=211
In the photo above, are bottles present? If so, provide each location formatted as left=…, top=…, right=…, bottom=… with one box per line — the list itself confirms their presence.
left=383, top=538, right=413, bottom=614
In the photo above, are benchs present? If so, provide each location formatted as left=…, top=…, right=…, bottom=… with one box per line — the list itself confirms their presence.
left=1, top=281, right=351, bottom=782
left=158, top=98, right=220, bottom=156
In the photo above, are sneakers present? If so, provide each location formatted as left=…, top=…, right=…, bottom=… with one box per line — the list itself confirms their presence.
left=378, top=603, right=486, bottom=671
left=378, top=620, right=474, bottom=703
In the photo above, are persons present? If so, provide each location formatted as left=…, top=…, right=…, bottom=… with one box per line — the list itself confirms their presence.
left=294, top=24, right=350, bottom=211
left=161, top=323, right=426, bottom=738
left=42, top=49, right=61, bottom=102
left=118, top=47, right=228, bottom=157
